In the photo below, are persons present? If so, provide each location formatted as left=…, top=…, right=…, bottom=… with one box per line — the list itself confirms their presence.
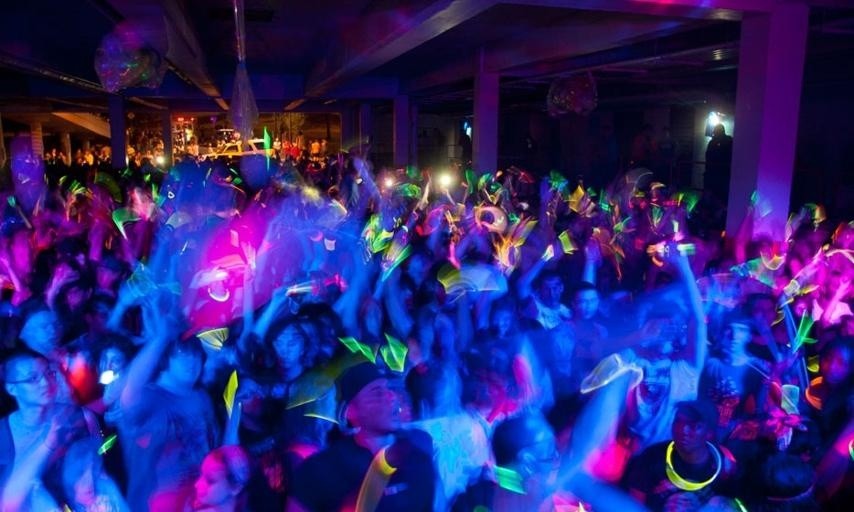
left=1, top=117, right=854, bottom=510
